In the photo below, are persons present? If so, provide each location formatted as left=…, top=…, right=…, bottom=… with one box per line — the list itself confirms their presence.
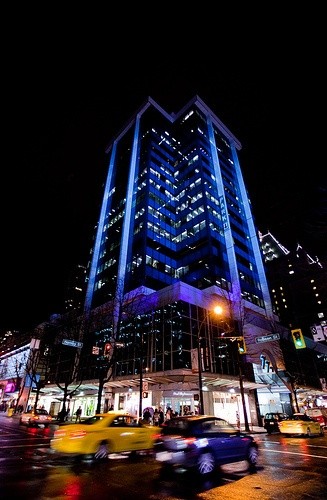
left=57, top=408, right=70, bottom=421
left=76, top=406, right=82, bottom=421
left=109, top=405, right=114, bottom=411
left=194, top=406, right=199, bottom=414
left=176, top=406, right=193, bottom=416
left=153, top=407, right=175, bottom=426
left=0, top=399, right=44, bottom=413
left=143, top=408, right=151, bottom=421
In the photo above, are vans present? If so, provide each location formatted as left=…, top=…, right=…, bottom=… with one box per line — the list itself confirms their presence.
left=263, top=412, right=288, bottom=434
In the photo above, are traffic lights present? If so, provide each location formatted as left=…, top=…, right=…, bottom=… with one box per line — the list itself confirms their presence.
left=142, top=392, right=148, bottom=399
left=291, top=329, right=306, bottom=349
left=237, top=337, right=247, bottom=355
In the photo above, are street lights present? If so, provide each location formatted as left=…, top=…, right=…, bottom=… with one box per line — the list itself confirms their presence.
left=198, top=304, right=223, bottom=415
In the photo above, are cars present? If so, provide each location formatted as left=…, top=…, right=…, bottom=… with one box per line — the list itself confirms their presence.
left=19, top=409, right=52, bottom=426
left=278, top=413, right=324, bottom=438
left=152, top=415, right=260, bottom=476
left=49, top=412, right=163, bottom=463
